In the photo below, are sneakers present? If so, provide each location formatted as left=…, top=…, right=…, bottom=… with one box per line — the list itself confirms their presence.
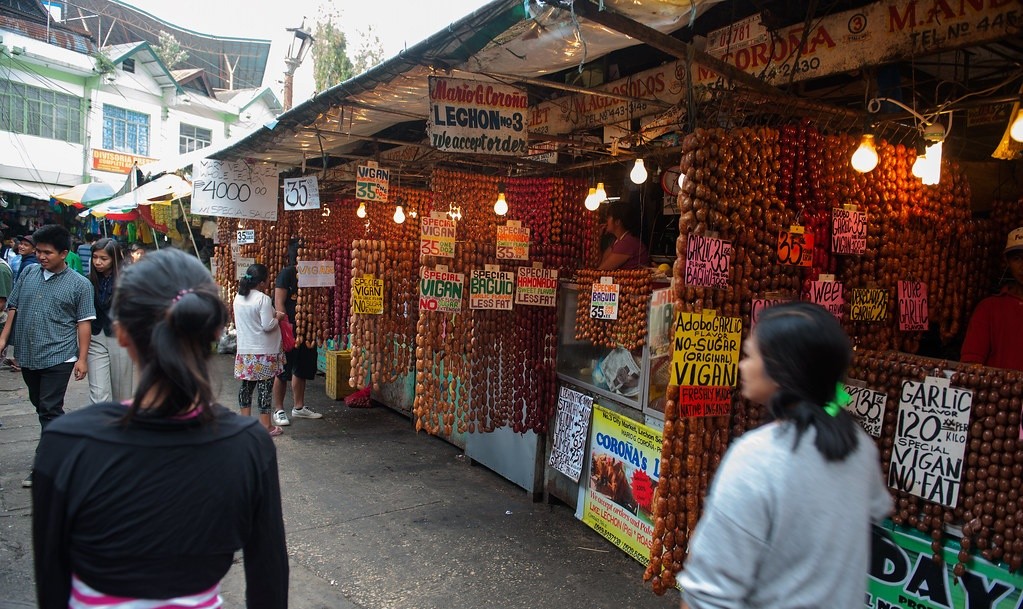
left=273, top=408, right=290, bottom=425
left=21, top=472, right=32, bottom=486
left=291, top=406, right=322, bottom=419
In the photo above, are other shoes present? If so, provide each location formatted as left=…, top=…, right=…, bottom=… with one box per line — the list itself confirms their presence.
left=3, top=358, right=21, bottom=371
left=269, top=426, right=284, bottom=436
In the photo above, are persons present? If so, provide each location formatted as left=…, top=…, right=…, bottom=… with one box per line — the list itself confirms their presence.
left=30, top=244, right=289, bottom=609
left=82, top=236, right=134, bottom=405
left=273, top=236, right=324, bottom=425
left=1, top=221, right=99, bottom=373
left=958, top=224, right=1023, bottom=373
left=0, top=222, right=97, bottom=486
left=673, top=300, right=895, bottom=609
left=233, top=262, right=287, bottom=436
left=595, top=201, right=650, bottom=272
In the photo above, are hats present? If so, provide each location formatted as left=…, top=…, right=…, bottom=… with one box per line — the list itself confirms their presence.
left=16, top=235, right=36, bottom=247
left=1002, top=227, right=1023, bottom=255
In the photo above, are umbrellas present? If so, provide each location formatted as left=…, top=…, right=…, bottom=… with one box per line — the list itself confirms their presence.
left=50, top=171, right=206, bottom=265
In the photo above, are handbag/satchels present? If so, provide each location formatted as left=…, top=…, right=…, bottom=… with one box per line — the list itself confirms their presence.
left=280, top=313, right=296, bottom=353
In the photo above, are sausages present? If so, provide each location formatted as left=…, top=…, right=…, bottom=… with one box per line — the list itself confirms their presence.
left=640, top=110, right=1022, bottom=607
left=209, top=168, right=653, bottom=443
